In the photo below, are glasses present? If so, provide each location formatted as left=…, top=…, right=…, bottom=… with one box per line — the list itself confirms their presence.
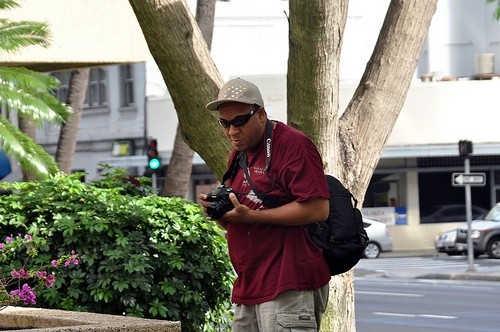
left=217, top=106, right=259, bottom=128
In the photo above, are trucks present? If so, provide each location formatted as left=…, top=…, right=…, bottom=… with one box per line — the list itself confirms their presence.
left=373, top=174, right=407, bottom=210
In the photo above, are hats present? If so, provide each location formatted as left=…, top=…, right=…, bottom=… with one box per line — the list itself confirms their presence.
left=205, top=76, right=266, bottom=112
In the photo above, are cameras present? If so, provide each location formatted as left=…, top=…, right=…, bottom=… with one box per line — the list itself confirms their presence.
left=205, top=185, right=241, bottom=220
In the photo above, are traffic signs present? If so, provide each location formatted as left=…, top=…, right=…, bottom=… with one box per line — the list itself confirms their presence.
left=453, top=172, right=486, bottom=189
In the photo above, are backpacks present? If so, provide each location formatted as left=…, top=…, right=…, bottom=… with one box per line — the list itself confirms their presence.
left=236, top=148, right=371, bottom=276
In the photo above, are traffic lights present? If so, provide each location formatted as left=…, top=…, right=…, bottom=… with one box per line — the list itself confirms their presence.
left=148, top=139, right=160, bottom=173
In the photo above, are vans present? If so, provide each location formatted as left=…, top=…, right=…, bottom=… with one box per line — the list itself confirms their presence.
left=457, top=203, right=500, bottom=259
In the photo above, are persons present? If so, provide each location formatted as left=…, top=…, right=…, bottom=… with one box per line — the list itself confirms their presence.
left=198, top=78, right=330, bottom=332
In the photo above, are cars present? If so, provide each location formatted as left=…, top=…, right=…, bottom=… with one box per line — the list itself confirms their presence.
left=359, top=217, right=392, bottom=260
left=436, top=217, right=483, bottom=257
left=421, top=205, right=488, bottom=235
